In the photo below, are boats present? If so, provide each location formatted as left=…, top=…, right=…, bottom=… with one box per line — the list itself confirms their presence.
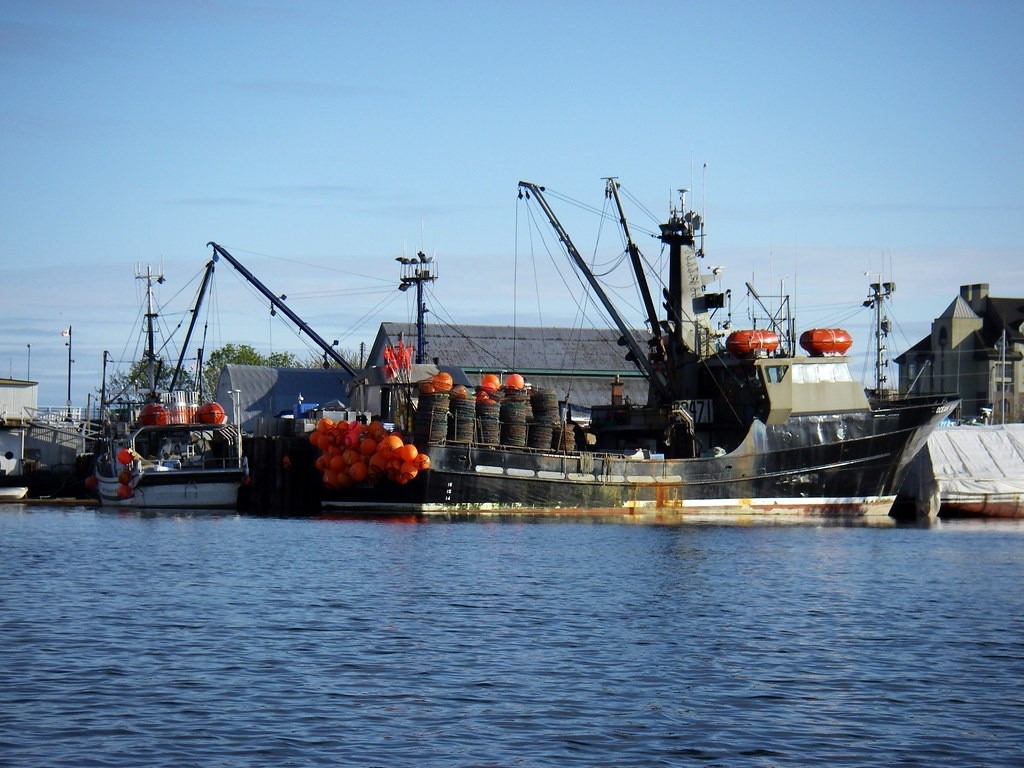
left=85, top=152, right=1023, bottom=520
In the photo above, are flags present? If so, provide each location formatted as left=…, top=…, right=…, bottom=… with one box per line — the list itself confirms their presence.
left=384, top=337, right=413, bottom=378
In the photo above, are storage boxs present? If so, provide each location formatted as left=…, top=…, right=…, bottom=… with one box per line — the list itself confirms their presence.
left=293, top=404, right=319, bottom=432
left=316, top=410, right=372, bottom=425
